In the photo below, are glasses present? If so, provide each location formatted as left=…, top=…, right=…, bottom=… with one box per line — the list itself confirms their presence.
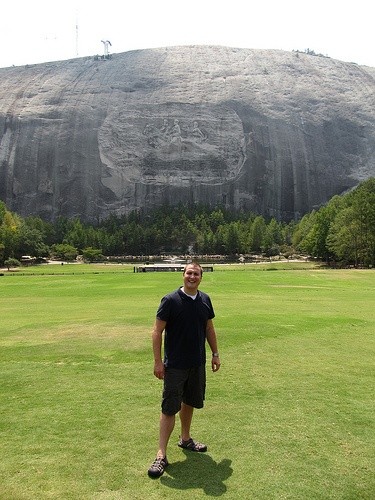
left=185, top=272, right=202, bottom=277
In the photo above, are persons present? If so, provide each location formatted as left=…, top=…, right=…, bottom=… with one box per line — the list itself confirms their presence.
left=146, top=261, right=222, bottom=478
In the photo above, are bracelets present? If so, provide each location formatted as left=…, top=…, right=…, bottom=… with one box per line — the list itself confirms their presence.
left=213, top=353, right=219, bottom=356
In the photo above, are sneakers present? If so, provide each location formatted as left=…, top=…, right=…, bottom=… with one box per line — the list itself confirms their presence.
left=179, top=438, right=207, bottom=452
left=148, top=456, right=168, bottom=478
